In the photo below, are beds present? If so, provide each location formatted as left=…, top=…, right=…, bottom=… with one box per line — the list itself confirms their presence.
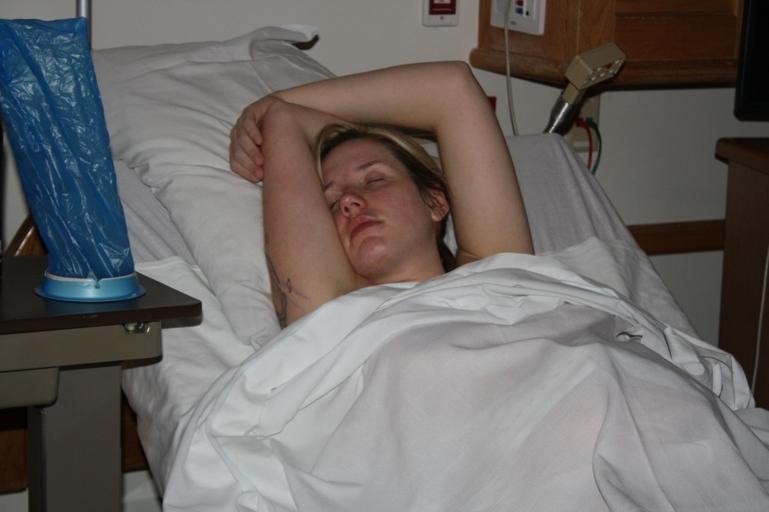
left=0, top=132, right=769, bottom=512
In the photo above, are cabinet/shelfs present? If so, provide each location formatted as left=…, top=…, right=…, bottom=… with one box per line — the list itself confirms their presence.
left=469, top=0, right=744, bottom=90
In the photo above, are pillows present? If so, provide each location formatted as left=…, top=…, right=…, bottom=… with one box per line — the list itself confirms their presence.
left=88, top=23, right=459, bottom=356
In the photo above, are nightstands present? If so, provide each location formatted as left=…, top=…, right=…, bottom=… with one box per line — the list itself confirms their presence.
left=714, top=137, right=769, bottom=412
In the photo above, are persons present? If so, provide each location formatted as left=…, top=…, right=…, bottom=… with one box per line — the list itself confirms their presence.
left=229, top=61, right=535, bottom=329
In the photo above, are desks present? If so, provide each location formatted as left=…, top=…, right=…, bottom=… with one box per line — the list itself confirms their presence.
left=0, top=254, right=203, bottom=511
